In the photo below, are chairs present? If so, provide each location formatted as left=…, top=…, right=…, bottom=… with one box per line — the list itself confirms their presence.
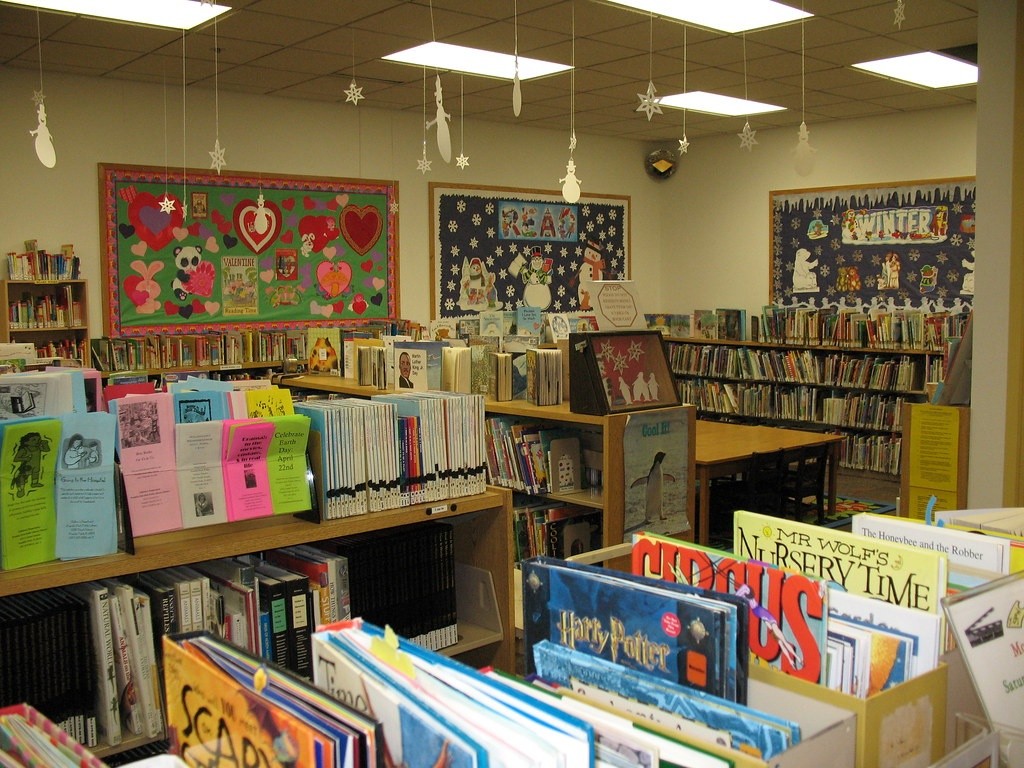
left=711, top=442, right=830, bottom=533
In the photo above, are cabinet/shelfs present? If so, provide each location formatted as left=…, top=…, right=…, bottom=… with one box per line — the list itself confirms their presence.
left=269, top=373, right=697, bottom=656
left=0, top=279, right=91, bottom=368
left=663, top=335, right=945, bottom=483
left=101, top=358, right=308, bottom=400
left=0, top=484, right=516, bottom=753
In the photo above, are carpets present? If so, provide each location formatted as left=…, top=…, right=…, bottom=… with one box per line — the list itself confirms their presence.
left=694, top=490, right=896, bottom=553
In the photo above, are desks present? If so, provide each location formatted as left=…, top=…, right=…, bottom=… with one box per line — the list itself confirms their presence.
left=696, top=419, right=847, bottom=546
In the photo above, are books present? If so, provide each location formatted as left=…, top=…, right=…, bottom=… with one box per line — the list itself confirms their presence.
left=0, top=240, right=1024, bottom=768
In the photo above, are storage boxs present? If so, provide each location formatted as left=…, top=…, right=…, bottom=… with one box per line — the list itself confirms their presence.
left=899, top=486, right=968, bottom=521
left=749, top=660, right=948, bottom=768
left=747, top=678, right=858, bottom=768
left=900, top=402, right=970, bottom=492
